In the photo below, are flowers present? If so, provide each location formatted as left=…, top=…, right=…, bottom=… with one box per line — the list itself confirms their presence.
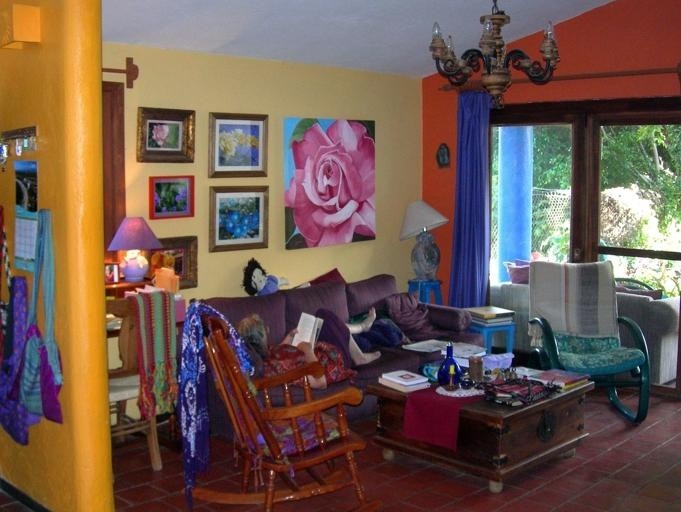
left=150, top=123, right=168, bottom=147
left=219, top=127, right=260, bottom=159
left=282, top=117, right=377, bottom=250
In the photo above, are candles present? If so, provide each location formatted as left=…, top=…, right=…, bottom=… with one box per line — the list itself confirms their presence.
left=448, top=363, right=456, bottom=374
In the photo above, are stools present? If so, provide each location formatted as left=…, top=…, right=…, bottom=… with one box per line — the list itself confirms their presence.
left=405, top=277, right=445, bottom=306
left=465, top=320, right=519, bottom=356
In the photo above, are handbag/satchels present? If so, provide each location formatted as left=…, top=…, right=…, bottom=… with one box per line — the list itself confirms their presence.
left=485, top=380, right=547, bottom=408
left=8, top=324, right=63, bottom=424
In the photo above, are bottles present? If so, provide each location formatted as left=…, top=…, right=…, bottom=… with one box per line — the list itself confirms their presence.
left=438, top=341, right=461, bottom=391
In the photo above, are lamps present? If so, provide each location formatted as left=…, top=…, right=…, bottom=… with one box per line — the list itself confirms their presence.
left=107, top=217, right=164, bottom=282
left=401, top=200, right=450, bottom=280
left=429, top=1, right=562, bottom=110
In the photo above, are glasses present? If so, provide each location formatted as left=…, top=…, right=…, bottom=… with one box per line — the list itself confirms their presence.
left=461, top=379, right=486, bottom=389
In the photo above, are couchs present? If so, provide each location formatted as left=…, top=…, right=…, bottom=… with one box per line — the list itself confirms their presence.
left=491, top=280, right=681, bottom=385
left=198, top=274, right=483, bottom=441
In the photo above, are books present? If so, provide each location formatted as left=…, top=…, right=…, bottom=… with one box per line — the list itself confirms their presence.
left=508, top=366, right=545, bottom=380
left=463, top=305, right=517, bottom=319
left=442, top=342, right=488, bottom=367
left=291, top=311, right=325, bottom=353
left=471, top=315, right=514, bottom=324
left=378, top=376, right=432, bottom=393
left=530, top=369, right=590, bottom=386
left=558, top=379, right=588, bottom=392
left=382, top=369, right=430, bottom=386
left=470, top=321, right=514, bottom=328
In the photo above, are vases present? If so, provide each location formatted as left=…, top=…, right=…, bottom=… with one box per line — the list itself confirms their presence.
left=438, top=341, right=464, bottom=386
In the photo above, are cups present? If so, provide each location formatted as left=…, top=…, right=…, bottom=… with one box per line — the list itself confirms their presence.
left=468, top=355, right=483, bottom=382
left=424, top=364, right=438, bottom=382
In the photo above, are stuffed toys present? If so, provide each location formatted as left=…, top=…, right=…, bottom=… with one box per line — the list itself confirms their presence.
left=239, top=255, right=312, bottom=297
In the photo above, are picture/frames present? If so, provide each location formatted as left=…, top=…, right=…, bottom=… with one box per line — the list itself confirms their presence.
left=149, top=176, right=195, bottom=219
left=136, top=106, right=195, bottom=164
left=208, top=112, right=268, bottom=178
left=210, top=185, right=269, bottom=252
left=152, top=235, right=199, bottom=291
left=105, top=263, right=120, bottom=284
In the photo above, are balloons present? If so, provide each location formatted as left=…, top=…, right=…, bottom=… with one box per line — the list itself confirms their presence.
left=218, top=211, right=260, bottom=236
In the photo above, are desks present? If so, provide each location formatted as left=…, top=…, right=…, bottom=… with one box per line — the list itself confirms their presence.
left=105, top=281, right=155, bottom=298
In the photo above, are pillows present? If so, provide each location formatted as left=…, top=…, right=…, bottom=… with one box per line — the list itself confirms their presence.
left=384, top=290, right=431, bottom=335
left=503, top=258, right=662, bottom=300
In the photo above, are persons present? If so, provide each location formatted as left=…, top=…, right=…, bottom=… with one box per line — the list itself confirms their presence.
left=237, top=307, right=384, bottom=391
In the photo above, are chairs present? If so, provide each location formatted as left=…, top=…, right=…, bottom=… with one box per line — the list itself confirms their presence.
left=106, top=293, right=165, bottom=474
left=182, top=303, right=367, bottom=511
left=529, top=260, right=651, bottom=442
